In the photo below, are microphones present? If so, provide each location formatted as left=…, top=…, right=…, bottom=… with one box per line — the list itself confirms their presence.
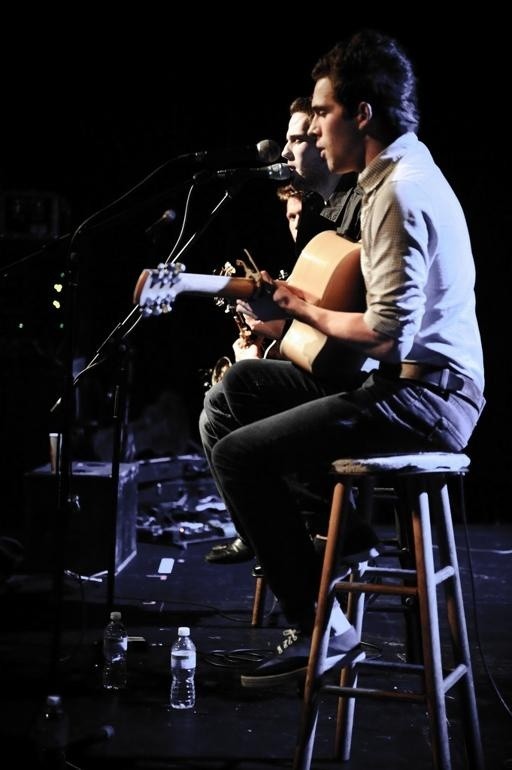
left=216, top=162, right=289, bottom=181
left=197, top=138, right=278, bottom=164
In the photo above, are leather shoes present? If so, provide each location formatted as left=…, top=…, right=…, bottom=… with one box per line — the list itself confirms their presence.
left=202, top=537, right=255, bottom=566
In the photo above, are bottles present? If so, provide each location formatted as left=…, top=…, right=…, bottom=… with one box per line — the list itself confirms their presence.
left=169, top=625, right=197, bottom=711
left=100, top=611, right=130, bottom=692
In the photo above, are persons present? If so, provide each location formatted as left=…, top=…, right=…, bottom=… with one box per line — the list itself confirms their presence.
left=211, top=30, right=488, bottom=692
left=231, top=183, right=303, bottom=360
left=190, top=97, right=366, bottom=564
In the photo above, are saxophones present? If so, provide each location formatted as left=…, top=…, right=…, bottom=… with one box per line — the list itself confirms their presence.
left=211, top=259, right=272, bottom=363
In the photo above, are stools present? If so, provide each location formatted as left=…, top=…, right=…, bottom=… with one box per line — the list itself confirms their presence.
left=332, top=485, right=417, bottom=658
left=294, top=451, right=484, bottom=770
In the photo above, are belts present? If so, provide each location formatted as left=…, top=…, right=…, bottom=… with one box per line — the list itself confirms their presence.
left=399, top=363, right=486, bottom=415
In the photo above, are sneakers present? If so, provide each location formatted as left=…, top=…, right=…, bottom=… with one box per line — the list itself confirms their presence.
left=240, top=624, right=366, bottom=689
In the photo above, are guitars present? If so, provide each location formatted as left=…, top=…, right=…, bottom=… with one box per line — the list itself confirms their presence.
left=134, top=229, right=360, bottom=375
